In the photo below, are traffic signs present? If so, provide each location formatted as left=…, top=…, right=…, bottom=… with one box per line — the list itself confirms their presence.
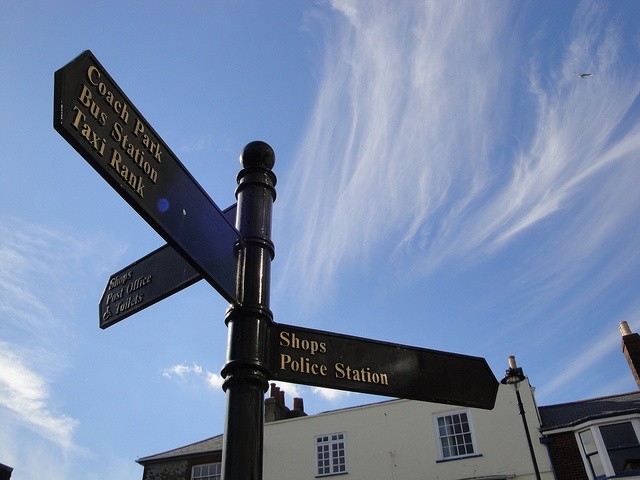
left=98, top=203, right=236, bottom=329
left=53, top=50, right=239, bottom=307
left=269, top=322, right=499, bottom=410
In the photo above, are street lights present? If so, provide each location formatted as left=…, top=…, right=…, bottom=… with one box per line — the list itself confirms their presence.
left=501, top=367, right=541, bottom=480
